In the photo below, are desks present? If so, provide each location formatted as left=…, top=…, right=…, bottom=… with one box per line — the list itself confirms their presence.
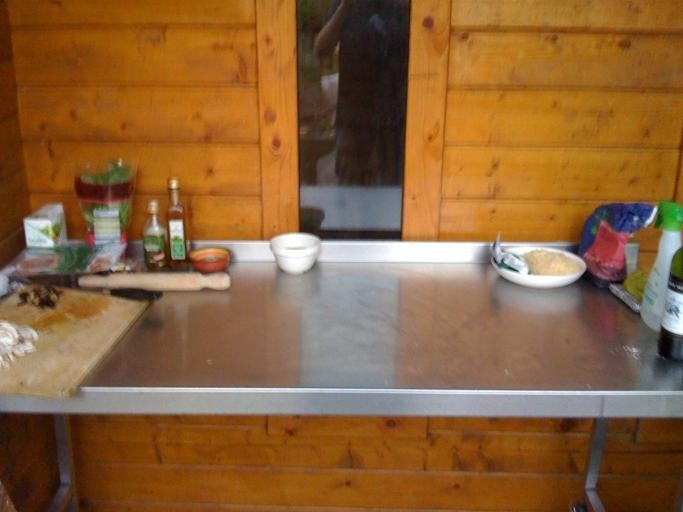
left=0, top=239, right=683, bottom=512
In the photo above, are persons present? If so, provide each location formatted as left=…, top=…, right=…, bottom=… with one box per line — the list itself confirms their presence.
left=305, top=1, right=399, bottom=184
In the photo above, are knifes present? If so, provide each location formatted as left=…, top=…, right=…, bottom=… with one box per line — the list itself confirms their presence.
left=82, top=284, right=163, bottom=302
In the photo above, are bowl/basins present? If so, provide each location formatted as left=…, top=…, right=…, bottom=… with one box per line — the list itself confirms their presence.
left=269, top=228, right=322, bottom=275
left=192, top=246, right=233, bottom=273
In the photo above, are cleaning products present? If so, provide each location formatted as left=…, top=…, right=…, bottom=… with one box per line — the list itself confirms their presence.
left=638, top=200, right=683, bottom=335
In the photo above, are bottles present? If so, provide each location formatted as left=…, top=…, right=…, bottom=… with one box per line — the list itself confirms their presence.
left=139, top=178, right=190, bottom=273
left=658, top=245, right=683, bottom=364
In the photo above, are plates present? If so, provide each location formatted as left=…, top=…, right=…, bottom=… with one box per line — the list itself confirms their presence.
left=488, top=244, right=588, bottom=287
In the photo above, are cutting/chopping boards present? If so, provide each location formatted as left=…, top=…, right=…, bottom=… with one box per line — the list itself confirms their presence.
left=0, top=275, right=150, bottom=400
